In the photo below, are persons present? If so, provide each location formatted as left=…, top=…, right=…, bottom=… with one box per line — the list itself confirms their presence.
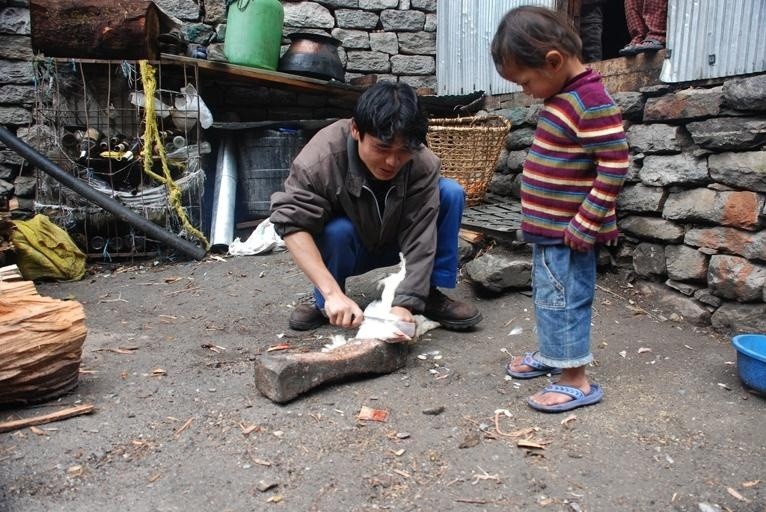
left=486, top=5, right=631, bottom=417
left=266, top=77, right=484, bottom=333
left=616, top=0, right=667, bottom=55
left=580, top=1, right=607, bottom=64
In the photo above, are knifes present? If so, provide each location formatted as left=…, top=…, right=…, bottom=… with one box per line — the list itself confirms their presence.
left=353, top=314, right=417, bottom=339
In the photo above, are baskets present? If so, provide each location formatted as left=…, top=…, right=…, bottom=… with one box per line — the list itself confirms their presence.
left=422, top=112, right=512, bottom=207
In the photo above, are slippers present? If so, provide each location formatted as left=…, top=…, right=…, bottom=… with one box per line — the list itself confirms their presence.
left=636, top=39, right=666, bottom=50
left=506, top=345, right=597, bottom=381
left=618, top=40, right=638, bottom=54
left=529, top=379, right=602, bottom=412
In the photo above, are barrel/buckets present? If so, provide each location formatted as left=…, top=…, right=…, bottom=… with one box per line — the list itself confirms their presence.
left=223, top=0, right=284, bottom=71
left=237, top=136, right=305, bottom=222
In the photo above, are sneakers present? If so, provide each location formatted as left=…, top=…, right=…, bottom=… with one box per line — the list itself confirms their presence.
left=408, top=285, right=481, bottom=330
left=287, top=286, right=346, bottom=332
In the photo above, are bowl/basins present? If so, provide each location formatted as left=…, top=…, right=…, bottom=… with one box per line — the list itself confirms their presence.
left=278, top=32, right=349, bottom=82
left=732, top=332, right=765, bottom=394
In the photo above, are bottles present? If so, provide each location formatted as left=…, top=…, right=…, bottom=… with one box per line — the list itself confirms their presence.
left=90, top=237, right=124, bottom=252
left=63, top=126, right=185, bottom=161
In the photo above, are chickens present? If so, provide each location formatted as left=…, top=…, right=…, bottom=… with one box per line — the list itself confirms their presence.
left=319, top=253, right=442, bottom=351
